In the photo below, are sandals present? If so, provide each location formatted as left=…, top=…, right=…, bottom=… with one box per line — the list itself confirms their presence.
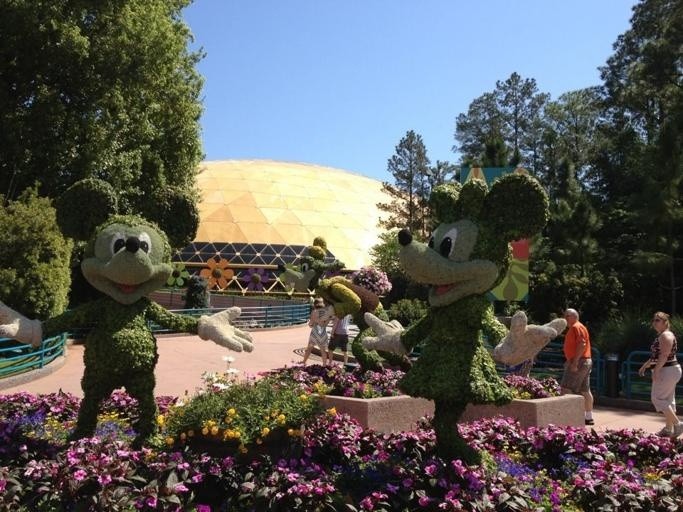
left=585, top=418, right=594, bottom=425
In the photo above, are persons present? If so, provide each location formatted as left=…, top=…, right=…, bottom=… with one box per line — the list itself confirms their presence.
left=301, top=296, right=329, bottom=368
left=324, top=315, right=351, bottom=370
left=637, top=310, right=683, bottom=441
left=560, top=308, right=597, bottom=427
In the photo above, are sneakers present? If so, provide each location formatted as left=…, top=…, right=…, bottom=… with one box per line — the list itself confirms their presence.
left=655, top=427, right=672, bottom=437
left=672, top=421, right=683, bottom=438
